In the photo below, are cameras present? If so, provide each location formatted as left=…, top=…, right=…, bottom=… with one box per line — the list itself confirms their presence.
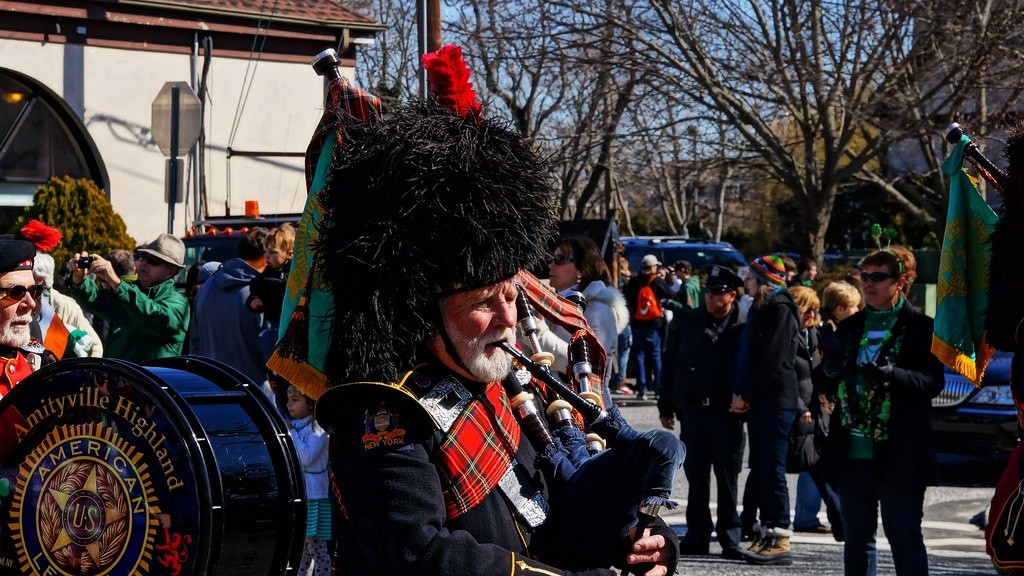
left=77, top=257, right=93, bottom=268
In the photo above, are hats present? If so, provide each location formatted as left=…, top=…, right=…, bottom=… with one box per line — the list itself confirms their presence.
left=671, top=261, right=690, bottom=272
left=137, top=233, right=187, bottom=268
left=20, top=219, right=63, bottom=289
left=620, top=257, right=631, bottom=276
left=641, top=254, right=662, bottom=268
left=0, top=233, right=37, bottom=279
left=748, top=255, right=787, bottom=287
left=311, top=45, right=558, bottom=386
left=196, top=261, right=224, bottom=283
left=698, top=265, right=745, bottom=294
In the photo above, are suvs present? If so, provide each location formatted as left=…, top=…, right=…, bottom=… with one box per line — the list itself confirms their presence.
left=618, top=237, right=747, bottom=274
left=921, top=348, right=1020, bottom=483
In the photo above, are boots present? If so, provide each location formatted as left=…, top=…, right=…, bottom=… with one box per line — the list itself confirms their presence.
left=736, top=524, right=793, bottom=563
left=297, top=540, right=312, bottom=576
left=307, top=539, right=331, bottom=576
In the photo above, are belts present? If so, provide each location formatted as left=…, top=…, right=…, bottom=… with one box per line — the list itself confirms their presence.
left=701, top=396, right=713, bottom=407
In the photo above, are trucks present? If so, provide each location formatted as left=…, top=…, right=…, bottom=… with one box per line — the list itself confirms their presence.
left=136, top=203, right=306, bottom=300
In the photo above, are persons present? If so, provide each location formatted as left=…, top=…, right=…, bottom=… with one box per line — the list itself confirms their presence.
left=657, top=265, right=748, bottom=558
left=260, top=371, right=286, bottom=422
left=786, top=280, right=846, bottom=534
left=311, top=104, right=674, bottom=576
left=285, top=382, right=331, bottom=576
left=984, top=132, right=1024, bottom=576
left=609, top=254, right=927, bottom=401
left=520, top=239, right=627, bottom=413
left=811, top=246, right=945, bottom=576
left=0, top=238, right=37, bottom=502
left=731, top=255, right=800, bottom=566
left=29, top=250, right=104, bottom=372
left=64, top=223, right=308, bottom=369
left=821, top=282, right=862, bottom=377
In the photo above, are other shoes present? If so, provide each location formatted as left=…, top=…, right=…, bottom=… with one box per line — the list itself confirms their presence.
left=637, top=394, right=647, bottom=400
left=719, top=547, right=741, bottom=560
left=678, top=540, right=709, bottom=556
left=616, top=382, right=634, bottom=395
left=793, top=522, right=832, bottom=533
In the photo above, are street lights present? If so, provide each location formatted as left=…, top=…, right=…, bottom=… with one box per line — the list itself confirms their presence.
left=687, top=125, right=698, bottom=218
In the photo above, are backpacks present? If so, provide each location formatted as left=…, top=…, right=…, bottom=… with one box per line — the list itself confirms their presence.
left=633, top=273, right=664, bottom=321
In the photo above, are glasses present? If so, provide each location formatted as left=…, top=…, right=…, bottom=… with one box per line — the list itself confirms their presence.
left=862, top=272, right=895, bottom=284
left=138, top=254, right=167, bottom=266
left=0, top=284, right=44, bottom=301
left=546, top=252, right=576, bottom=267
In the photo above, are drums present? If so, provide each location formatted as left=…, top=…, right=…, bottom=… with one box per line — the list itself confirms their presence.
left=0, top=356, right=309, bottom=576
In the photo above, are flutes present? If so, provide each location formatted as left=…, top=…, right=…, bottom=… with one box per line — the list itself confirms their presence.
left=309, top=44, right=689, bottom=573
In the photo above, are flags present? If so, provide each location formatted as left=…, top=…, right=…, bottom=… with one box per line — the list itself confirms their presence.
left=264, top=111, right=356, bottom=396
left=931, top=162, right=1001, bottom=387
